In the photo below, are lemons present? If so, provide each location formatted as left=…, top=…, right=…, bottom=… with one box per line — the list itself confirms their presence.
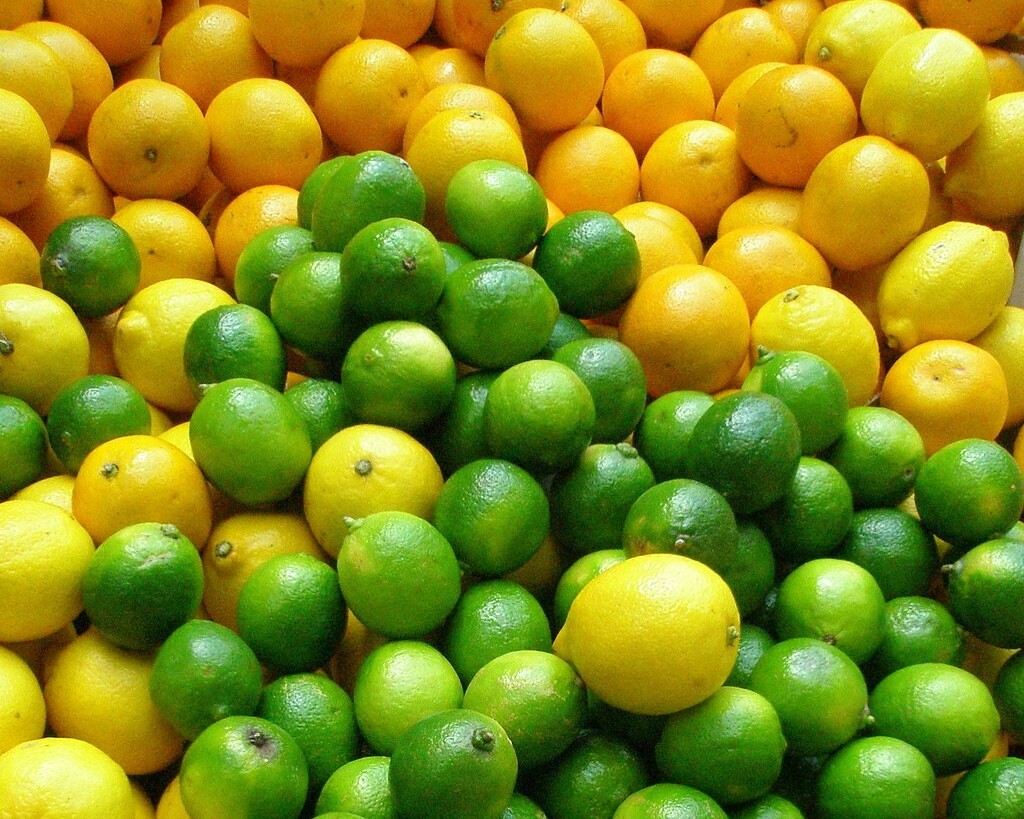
left=0, top=0, right=1024, bottom=819
